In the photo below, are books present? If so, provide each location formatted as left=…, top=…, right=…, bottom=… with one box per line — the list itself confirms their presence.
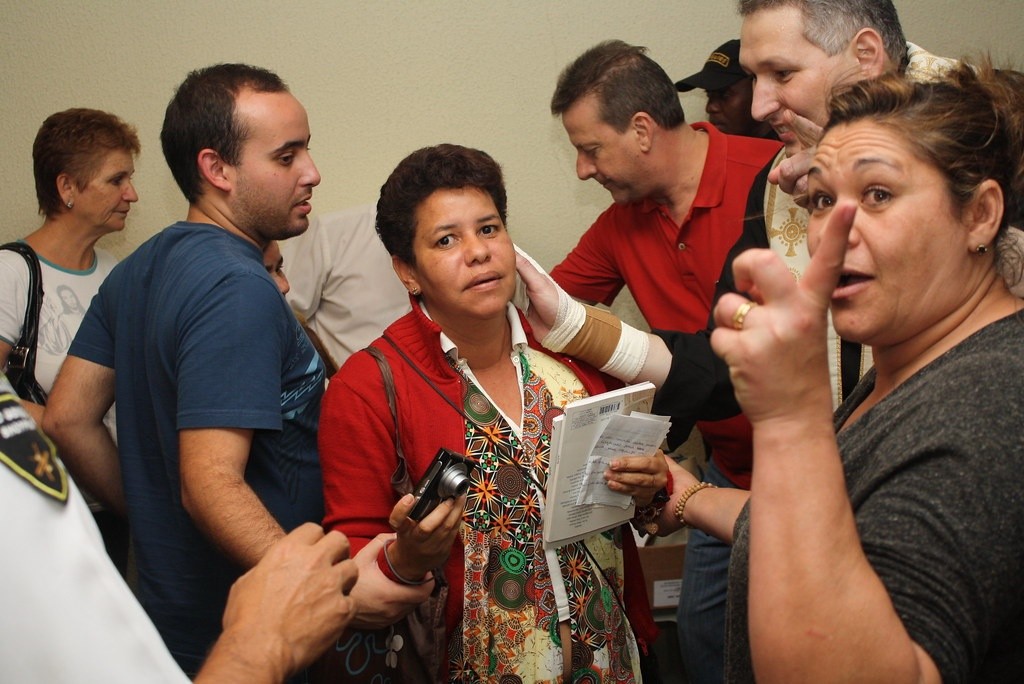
left=542, top=381, right=657, bottom=551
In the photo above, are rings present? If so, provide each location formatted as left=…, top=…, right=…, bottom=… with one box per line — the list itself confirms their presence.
left=732, top=301, right=759, bottom=329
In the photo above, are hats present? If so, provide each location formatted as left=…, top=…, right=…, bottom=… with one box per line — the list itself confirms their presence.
left=675, top=39, right=753, bottom=92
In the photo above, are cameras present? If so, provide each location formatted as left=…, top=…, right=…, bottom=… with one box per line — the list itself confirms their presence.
left=405, top=445, right=480, bottom=522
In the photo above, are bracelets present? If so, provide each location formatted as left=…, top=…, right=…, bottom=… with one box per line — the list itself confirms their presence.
left=674, top=481, right=719, bottom=529
left=630, top=472, right=674, bottom=538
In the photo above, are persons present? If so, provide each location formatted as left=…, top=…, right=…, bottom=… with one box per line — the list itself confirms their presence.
left=0, top=109, right=142, bottom=577
left=547, top=39, right=786, bottom=682
left=510, top=0, right=1023, bottom=411
left=646, top=60, right=1024, bottom=683
left=671, top=38, right=778, bottom=138
left=1, top=371, right=359, bottom=684
left=317, top=144, right=653, bottom=684
left=43, top=62, right=436, bottom=684
left=261, top=239, right=290, bottom=301
left=277, top=199, right=412, bottom=389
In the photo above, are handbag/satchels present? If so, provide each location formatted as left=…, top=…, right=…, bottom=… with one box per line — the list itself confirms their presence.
left=0, top=242, right=47, bottom=407
